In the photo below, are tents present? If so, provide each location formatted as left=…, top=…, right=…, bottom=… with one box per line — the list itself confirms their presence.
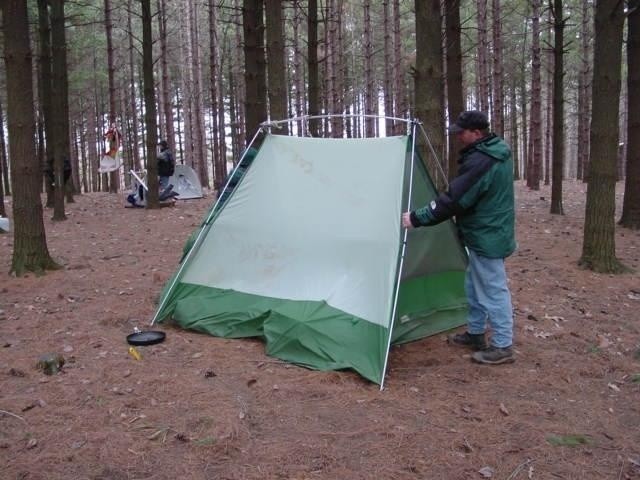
left=136, top=163, right=204, bottom=200
left=157, top=111, right=484, bottom=395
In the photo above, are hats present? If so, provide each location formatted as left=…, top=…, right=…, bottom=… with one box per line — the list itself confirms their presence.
left=448, top=111, right=489, bottom=132
left=157, top=140, right=167, bottom=147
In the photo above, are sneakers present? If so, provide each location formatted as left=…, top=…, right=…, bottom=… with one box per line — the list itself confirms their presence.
left=451, top=331, right=489, bottom=351
left=471, top=344, right=516, bottom=365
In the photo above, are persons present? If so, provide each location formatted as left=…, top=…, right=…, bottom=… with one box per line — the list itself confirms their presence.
left=155, top=140, right=174, bottom=189
left=402, top=111, right=518, bottom=366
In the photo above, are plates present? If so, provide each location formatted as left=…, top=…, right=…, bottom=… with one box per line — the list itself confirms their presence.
left=126, top=331, right=166, bottom=346
